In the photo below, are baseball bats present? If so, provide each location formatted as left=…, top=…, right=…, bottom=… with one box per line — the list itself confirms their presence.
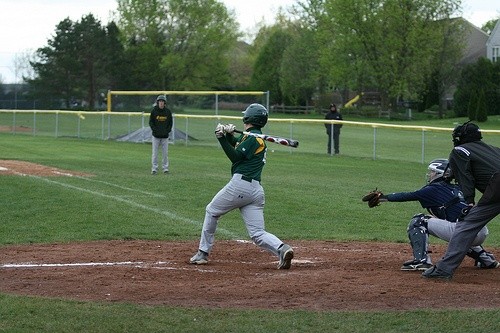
left=233, top=129, right=299, bottom=147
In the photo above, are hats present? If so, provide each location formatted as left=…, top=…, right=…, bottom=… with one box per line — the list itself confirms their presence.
left=329, top=105, right=337, bottom=113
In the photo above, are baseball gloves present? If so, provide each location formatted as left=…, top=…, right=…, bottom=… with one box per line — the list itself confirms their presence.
left=362, top=190, right=383, bottom=207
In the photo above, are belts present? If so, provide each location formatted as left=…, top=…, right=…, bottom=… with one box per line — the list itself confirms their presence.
left=241, top=176, right=252, bottom=183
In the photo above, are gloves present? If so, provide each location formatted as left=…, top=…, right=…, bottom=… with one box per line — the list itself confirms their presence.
left=223, top=123, right=235, bottom=135
left=460, top=206, right=472, bottom=218
left=215, top=123, right=225, bottom=138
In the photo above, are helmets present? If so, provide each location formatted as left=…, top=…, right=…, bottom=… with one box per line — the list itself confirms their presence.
left=157, top=95, right=167, bottom=102
left=453, top=122, right=480, bottom=146
left=427, top=159, right=452, bottom=183
left=242, top=104, right=268, bottom=127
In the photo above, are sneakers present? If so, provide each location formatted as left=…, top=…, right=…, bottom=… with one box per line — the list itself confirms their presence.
left=422, top=266, right=450, bottom=280
left=399, top=258, right=431, bottom=270
left=185, top=251, right=207, bottom=264
left=276, top=244, right=294, bottom=269
left=489, top=261, right=500, bottom=269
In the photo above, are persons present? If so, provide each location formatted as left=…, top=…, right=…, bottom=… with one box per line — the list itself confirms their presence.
left=324, top=104, right=343, bottom=155
left=189, top=104, right=295, bottom=270
left=421, top=122, right=500, bottom=279
left=361, top=158, right=500, bottom=271
left=149, top=95, right=172, bottom=175
